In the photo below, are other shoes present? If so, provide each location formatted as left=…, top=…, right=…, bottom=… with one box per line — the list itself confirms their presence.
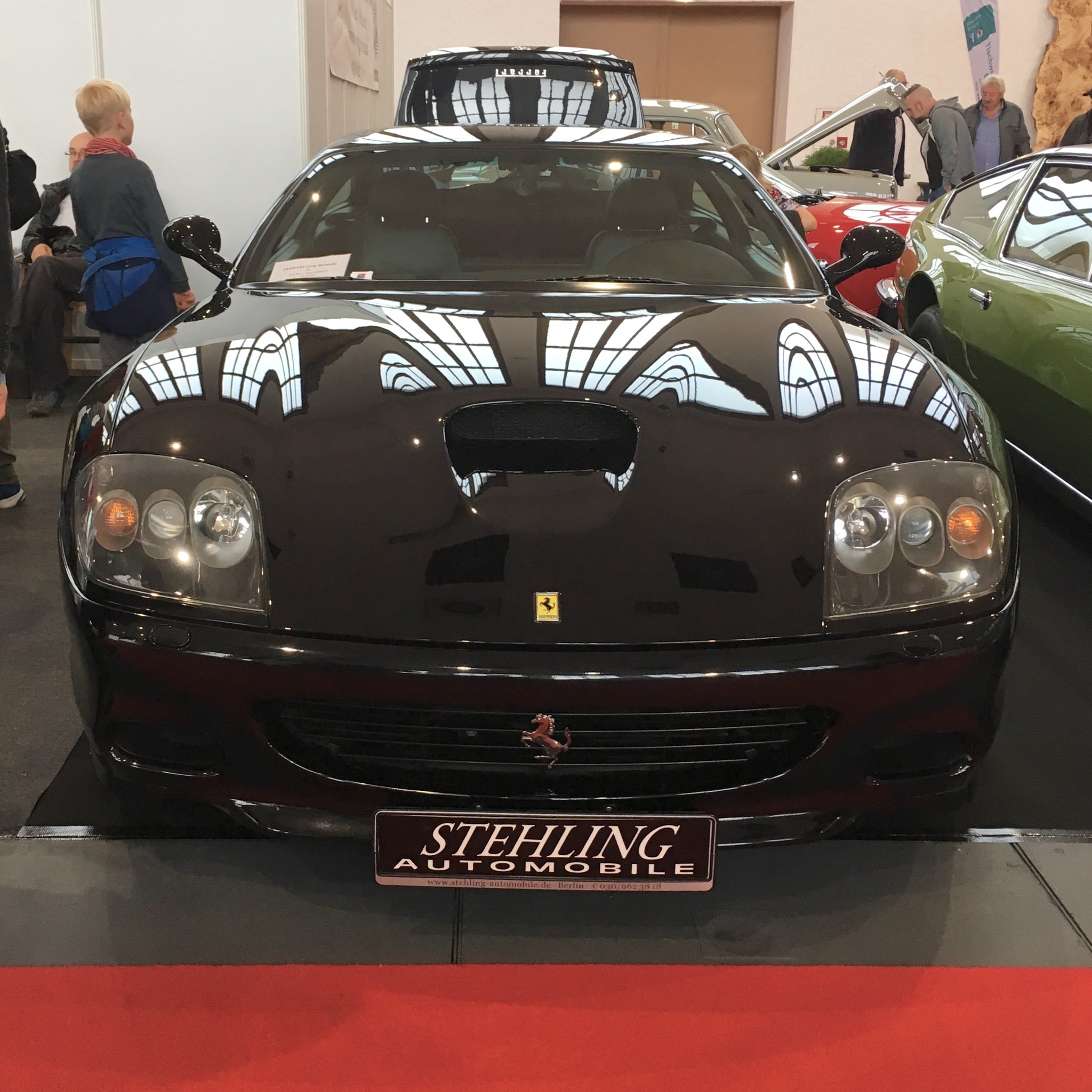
left=0, top=482, right=27, bottom=510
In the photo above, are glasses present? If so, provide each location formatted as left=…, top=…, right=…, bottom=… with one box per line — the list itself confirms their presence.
left=65, top=150, right=85, bottom=157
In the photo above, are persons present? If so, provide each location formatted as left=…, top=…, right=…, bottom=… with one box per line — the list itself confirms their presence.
left=0, top=118, right=97, bottom=508
left=904, top=74, right=1033, bottom=202
left=846, top=69, right=906, bottom=187
left=1060, top=89, right=1092, bottom=147
left=70, top=80, right=195, bottom=375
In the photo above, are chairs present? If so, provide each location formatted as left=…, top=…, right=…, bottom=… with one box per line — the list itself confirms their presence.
left=585, top=176, right=690, bottom=277
left=350, top=168, right=459, bottom=279
left=13, top=255, right=105, bottom=376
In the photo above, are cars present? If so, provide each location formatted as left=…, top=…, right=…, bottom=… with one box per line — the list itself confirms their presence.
left=638, top=80, right=931, bottom=327
left=899, top=143, right=1092, bottom=523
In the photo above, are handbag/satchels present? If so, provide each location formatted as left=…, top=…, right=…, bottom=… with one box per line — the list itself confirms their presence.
left=3, top=129, right=41, bottom=230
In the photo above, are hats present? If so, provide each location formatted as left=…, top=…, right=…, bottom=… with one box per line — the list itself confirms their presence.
left=1083, top=89, right=1092, bottom=98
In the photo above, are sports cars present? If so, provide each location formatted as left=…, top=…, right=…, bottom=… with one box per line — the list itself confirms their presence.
left=56, top=44, right=1020, bottom=893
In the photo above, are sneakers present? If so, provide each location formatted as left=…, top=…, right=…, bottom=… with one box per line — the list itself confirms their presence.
left=26, top=387, right=63, bottom=414
